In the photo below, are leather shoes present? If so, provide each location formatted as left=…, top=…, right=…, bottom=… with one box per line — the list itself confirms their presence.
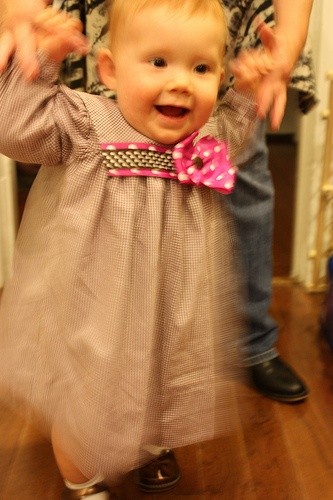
left=72, top=486, right=112, bottom=500
left=241, top=357, right=309, bottom=401
left=129, top=452, right=181, bottom=492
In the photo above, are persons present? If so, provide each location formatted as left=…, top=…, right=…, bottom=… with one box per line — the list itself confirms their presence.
left=0, top=1, right=274, bottom=500
left=0, top=0, right=319, bottom=404
